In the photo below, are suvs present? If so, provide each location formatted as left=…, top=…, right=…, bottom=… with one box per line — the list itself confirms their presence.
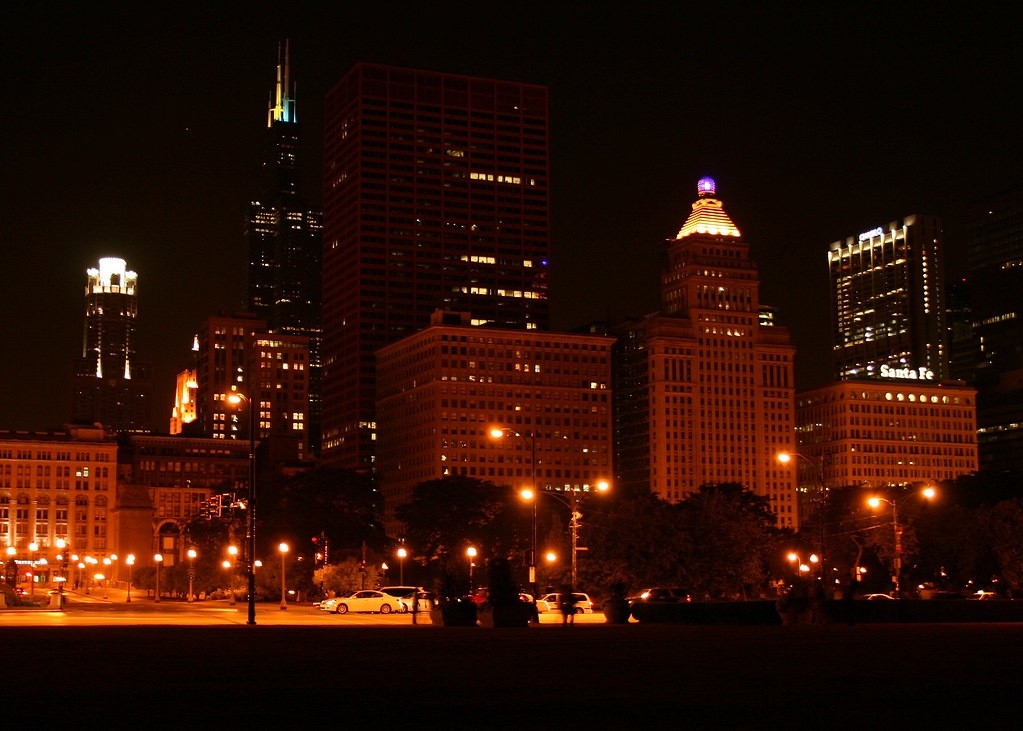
left=626, top=587, right=693, bottom=609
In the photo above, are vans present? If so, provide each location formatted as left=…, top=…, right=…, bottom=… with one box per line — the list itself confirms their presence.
left=372, top=585, right=427, bottom=614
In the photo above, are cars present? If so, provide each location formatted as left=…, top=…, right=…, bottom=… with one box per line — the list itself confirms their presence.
left=402, top=585, right=593, bottom=615
left=318, top=589, right=404, bottom=614
left=919, top=576, right=1015, bottom=601
left=867, top=593, right=900, bottom=600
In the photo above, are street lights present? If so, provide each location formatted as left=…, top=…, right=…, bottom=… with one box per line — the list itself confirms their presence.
left=397, top=548, right=407, bottom=586
left=29, top=542, right=39, bottom=605
left=788, top=553, right=801, bottom=577
left=490, top=426, right=538, bottom=624
left=85, top=555, right=91, bottom=594
left=467, top=547, right=477, bottom=583
left=868, top=497, right=900, bottom=600
left=522, top=481, right=609, bottom=592
left=154, top=553, right=164, bottom=602
left=57, top=539, right=65, bottom=611
left=126, top=554, right=135, bottom=602
left=809, top=554, right=823, bottom=581
left=779, top=453, right=826, bottom=601
left=77, top=563, right=85, bottom=589
left=228, top=546, right=238, bottom=606
left=227, top=391, right=257, bottom=624
left=185, top=549, right=197, bottom=603
left=279, top=542, right=289, bottom=609
left=102, top=557, right=112, bottom=599
